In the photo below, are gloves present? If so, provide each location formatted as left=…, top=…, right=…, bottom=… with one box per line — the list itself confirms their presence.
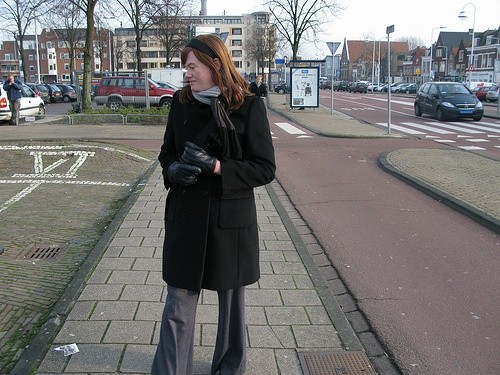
left=167, top=161, right=201, bottom=187
left=179, top=141, right=217, bottom=176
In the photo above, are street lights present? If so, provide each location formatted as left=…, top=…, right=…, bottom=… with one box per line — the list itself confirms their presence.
left=30, top=8, right=40, bottom=84
left=458, top=3, right=476, bottom=89
left=438, top=46, right=448, bottom=81
left=430, top=27, right=447, bottom=81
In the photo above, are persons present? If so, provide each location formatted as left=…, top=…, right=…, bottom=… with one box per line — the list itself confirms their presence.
left=150, top=33, right=276, bottom=375
left=249, top=74, right=268, bottom=98
left=3, top=73, right=24, bottom=126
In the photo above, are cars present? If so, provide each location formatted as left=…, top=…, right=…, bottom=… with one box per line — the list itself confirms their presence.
left=158, top=81, right=179, bottom=90
left=476, top=86, right=491, bottom=101
left=94, top=75, right=175, bottom=112
left=0, top=81, right=46, bottom=125
left=46, top=84, right=63, bottom=104
left=486, top=84, right=500, bottom=103
left=56, top=83, right=77, bottom=103
left=413, top=82, right=484, bottom=121
left=319, top=77, right=416, bottom=94
left=275, top=83, right=290, bottom=95
left=26, top=84, right=49, bottom=104
left=70, top=85, right=95, bottom=102
